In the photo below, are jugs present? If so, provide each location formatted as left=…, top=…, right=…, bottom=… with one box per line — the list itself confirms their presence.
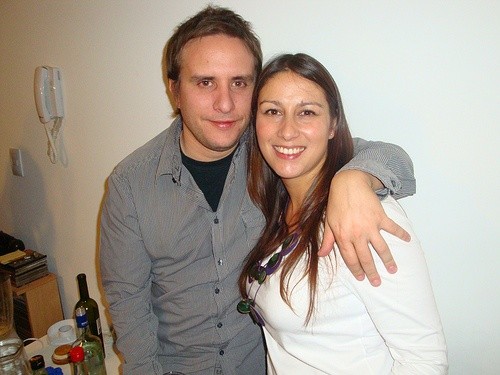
left=0, top=337, right=32, bottom=375
left=0, top=269, right=20, bottom=341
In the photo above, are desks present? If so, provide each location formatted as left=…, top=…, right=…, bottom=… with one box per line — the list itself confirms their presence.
left=12, top=272, right=64, bottom=343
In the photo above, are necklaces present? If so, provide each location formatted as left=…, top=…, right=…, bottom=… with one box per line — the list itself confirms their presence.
left=236, top=222, right=306, bottom=326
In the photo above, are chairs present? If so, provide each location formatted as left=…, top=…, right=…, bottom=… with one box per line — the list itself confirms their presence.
left=0, top=276, right=17, bottom=340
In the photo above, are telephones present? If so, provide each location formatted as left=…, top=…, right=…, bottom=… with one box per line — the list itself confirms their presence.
left=34, top=66, right=64, bottom=124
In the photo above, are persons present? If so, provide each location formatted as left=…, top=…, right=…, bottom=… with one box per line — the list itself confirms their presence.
left=99, top=4, right=416, bottom=375
left=238, top=54, right=449, bottom=375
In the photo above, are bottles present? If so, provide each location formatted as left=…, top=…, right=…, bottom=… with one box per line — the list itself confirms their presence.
left=71, top=306, right=107, bottom=375
left=69, top=346, right=89, bottom=375
left=74, top=273, right=106, bottom=359
left=29, top=355, right=49, bottom=375
